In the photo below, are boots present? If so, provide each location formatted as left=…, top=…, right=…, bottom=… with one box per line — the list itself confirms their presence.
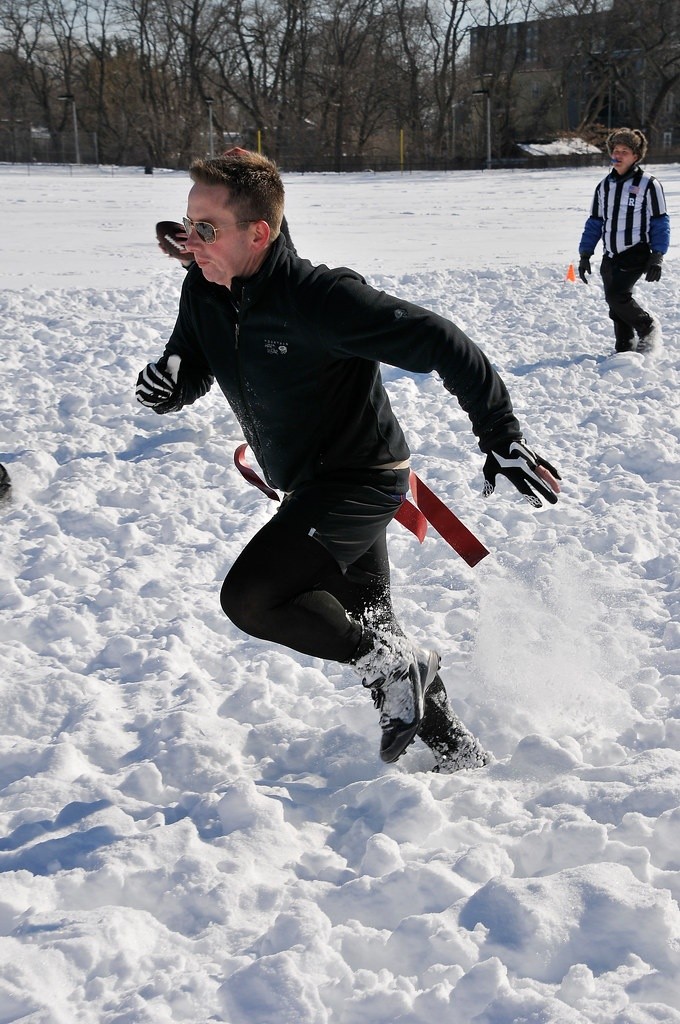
left=414, top=674, right=496, bottom=775
left=348, top=625, right=441, bottom=763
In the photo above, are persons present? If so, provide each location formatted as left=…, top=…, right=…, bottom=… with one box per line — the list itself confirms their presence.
left=135, top=149, right=562, bottom=775
left=579, top=128, right=670, bottom=355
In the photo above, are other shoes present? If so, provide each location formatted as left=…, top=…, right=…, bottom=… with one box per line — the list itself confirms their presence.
left=637, top=334, right=651, bottom=354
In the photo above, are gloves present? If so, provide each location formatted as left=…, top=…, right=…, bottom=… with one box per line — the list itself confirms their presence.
left=578, top=256, right=591, bottom=284
left=644, top=250, right=663, bottom=282
left=135, top=355, right=185, bottom=415
left=481, top=439, right=562, bottom=508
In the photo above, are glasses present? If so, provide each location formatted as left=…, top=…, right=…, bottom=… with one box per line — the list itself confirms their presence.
left=181, top=217, right=261, bottom=245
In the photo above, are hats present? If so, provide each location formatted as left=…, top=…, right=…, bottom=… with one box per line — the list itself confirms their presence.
left=606, top=128, right=648, bottom=166
